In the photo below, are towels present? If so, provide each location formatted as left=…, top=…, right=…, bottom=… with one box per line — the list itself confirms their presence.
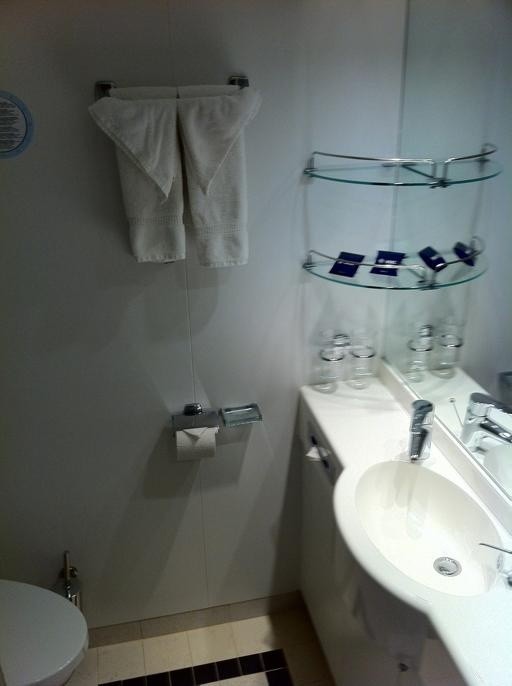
left=88, top=84, right=264, bottom=269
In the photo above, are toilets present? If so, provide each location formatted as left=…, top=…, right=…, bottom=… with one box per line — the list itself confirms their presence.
left=0, top=575, right=90, bottom=685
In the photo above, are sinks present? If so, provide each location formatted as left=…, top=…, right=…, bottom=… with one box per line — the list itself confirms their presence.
left=332, top=464, right=506, bottom=599
left=482, top=433, right=512, bottom=491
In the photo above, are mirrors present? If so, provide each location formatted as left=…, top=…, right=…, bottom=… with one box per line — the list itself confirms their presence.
left=377, top=0, right=512, bottom=536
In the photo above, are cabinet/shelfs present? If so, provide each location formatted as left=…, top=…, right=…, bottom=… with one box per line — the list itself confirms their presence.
left=401, top=143, right=504, bottom=289
left=301, top=151, right=432, bottom=290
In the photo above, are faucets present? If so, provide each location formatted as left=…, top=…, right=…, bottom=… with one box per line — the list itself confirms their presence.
left=463, top=391, right=511, bottom=444
left=408, top=400, right=434, bottom=463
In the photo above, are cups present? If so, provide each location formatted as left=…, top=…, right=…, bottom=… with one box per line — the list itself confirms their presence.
left=408, top=340, right=432, bottom=381
left=318, top=349, right=344, bottom=390
left=439, top=333, right=463, bottom=374
left=347, top=348, right=376, bottom=390
